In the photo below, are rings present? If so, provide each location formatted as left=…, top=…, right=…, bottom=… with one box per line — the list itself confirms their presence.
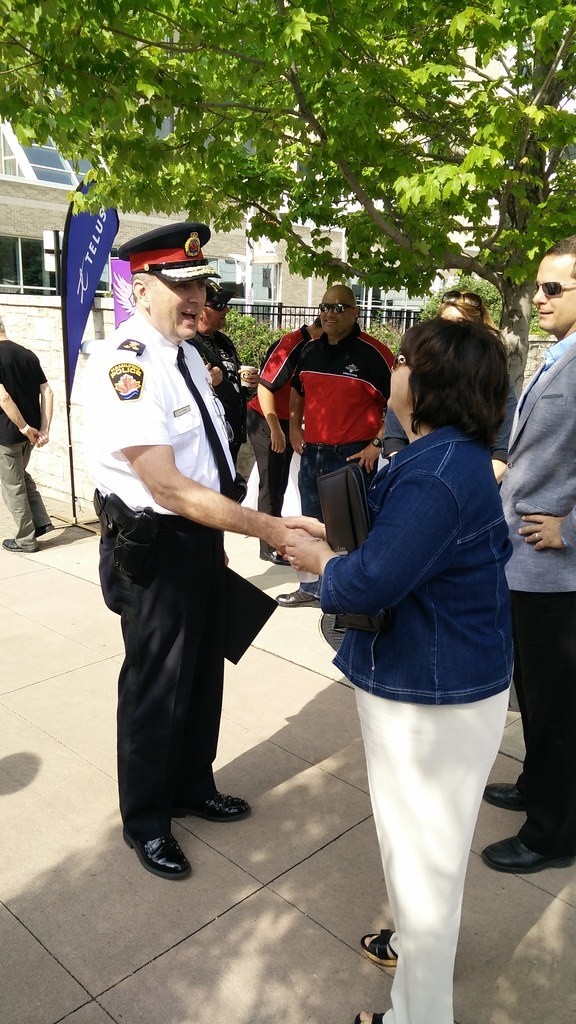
left=536, top=533, right=540, bottom=542
left=287, top=555, right=295, bottom=564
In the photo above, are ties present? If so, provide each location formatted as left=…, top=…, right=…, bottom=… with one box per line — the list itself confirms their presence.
left=176, top=346, right=234, bottom=498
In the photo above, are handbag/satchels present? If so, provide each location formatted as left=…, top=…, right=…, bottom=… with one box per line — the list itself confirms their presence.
left=316, top=462, right=391, bottom=633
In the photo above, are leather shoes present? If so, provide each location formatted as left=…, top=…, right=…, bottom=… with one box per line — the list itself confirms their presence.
left=170, top=786, right=251, bottom=824
left=2, top=537, right=38, bottom=553
left=36, top=522, right=55, bottom=536
left=260, top=548, right=291, bottom=564
left=122, top=819, right=190, bottom=879
left=276, top=587, right=322, bottom=607
left=483, top=782, right=528, bottom=811
left=482, top=829, right=576, bottom=874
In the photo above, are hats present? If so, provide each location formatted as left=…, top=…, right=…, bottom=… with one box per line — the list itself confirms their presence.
left=203, top=279, right=238, bottom=304
left=116, top=222, right=222, bottom=283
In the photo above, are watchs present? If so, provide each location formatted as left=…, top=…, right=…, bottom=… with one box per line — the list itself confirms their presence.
left=372, top=437, right=382, bottom=448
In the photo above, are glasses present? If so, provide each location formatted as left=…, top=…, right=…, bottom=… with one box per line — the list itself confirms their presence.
left=392, top=349, right=408, bottom=370
left=442, top=291, right=483, bottom=317
left=535, top=281, right=576, bottom=295
left=202, top=303, right=228, bottom=310
left=319, top=301, right=357, bottom=314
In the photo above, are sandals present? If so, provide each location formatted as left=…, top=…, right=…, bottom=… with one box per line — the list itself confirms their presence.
left=361, top=930, right=398, bottom=967
left=355, top=1012, right=385, bottom=1024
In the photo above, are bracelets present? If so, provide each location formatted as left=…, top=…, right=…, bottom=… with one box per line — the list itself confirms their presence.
left=19, top=424, right=30, bottom=434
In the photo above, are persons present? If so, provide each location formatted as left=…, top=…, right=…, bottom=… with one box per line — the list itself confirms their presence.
left=276, top=285, right=396, bottom=608
left=0, top=312, right=54, bottom=552
left=68, top=221, right=325, bottom=880
left=186, top=279, right=260, bottom=470
left=283, top=309, right=516, bottom=1024
left=383, top=290, right=518, bottom=492
left=481, top=237, right=576, bottom=874
left=247, top=315, right=323, bottom=567
